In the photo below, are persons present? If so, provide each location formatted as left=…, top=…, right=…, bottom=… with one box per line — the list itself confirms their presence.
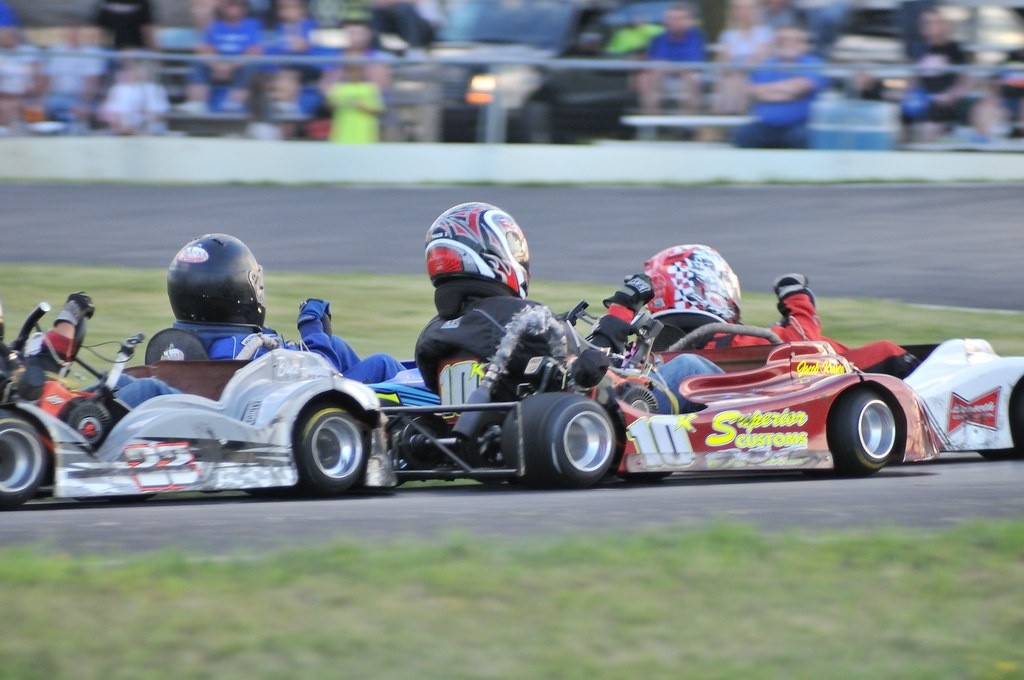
left=2, top=1, right=1023, bottom=150
left=414, top=201, right=655, bottom=403
left=166, top=232, right=408, bottom=386
left=643, top=244, right=908, bottom=372
left=0, top=290, right=186, bottom=426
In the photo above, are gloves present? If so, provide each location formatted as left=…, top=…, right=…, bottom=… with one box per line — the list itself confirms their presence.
left=603, top=273, right=655, bottom=319
left=772, top=273, right=808, bottom=301
left=297, top=298, right=331, bottom=329
left=54, top=291, right=94, bottom=328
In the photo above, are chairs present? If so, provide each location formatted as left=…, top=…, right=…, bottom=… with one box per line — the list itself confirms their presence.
left=649, top=323, right=697, bottom=352
left=144, top=327, right=209, bottom=364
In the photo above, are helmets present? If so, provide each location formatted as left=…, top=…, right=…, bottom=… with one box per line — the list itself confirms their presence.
left=424, top=202, right=530, bottom=301
left=167, top=233, right=266, bottom=331
left=644, top=245, right=742, bottom=325
